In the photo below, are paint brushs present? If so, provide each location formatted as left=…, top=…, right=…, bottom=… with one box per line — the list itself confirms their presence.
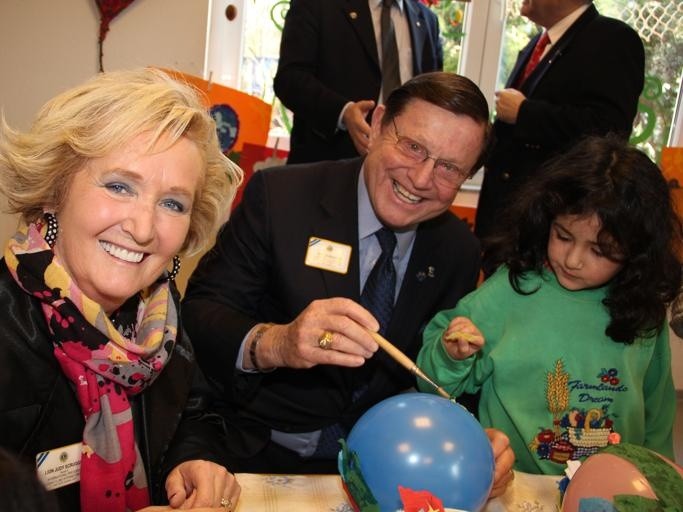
left=364, top=327, right=451, bottom=399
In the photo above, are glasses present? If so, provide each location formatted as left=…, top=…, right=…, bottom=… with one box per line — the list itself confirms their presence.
left=392, top=116, right=472, bottom=181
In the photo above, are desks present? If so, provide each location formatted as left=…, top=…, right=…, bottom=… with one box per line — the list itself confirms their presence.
left=232, top=470, right=567, bottom=512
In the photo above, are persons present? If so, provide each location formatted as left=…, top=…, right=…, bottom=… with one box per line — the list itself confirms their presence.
left=452, top=2, right=655, bottom=420
left=410, top=135, right=683, bottom=476
left=175, top=67, right=518, bottom=511
left=0, top=63, right=250, bottom=511
left=268, top=2, right=447, bottom=166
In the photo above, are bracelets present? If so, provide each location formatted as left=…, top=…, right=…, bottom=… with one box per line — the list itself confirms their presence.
left=246, top=321, right=280, bottom=374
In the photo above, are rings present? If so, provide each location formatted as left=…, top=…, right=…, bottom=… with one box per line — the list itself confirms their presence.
left=219, top=496, right=233, bottom=509
left=316, top=328, right=338, bottom=352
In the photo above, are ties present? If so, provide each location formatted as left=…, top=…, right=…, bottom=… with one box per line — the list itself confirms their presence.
left=513, top=31, right=551, bottom=90
left=381, top=0, right=401, bottom=105
left=311, top=227, right=397, bottom=474
